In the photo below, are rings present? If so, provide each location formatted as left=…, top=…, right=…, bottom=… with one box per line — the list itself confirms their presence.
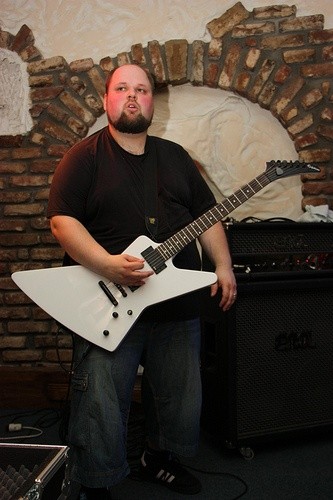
left=233, top=294, right=236, bottom=296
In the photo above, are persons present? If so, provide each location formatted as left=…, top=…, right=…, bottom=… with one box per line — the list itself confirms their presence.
left=47, top=63, right=237, bottom=499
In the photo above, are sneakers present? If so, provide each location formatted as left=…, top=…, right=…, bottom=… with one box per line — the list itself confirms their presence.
left=140, top=450, right=202, bottom=495
left=78, top=486, right=111, bottom=500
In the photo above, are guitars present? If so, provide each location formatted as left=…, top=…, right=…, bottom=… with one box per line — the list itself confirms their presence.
left=12, top=158, right=322, bottom=352
